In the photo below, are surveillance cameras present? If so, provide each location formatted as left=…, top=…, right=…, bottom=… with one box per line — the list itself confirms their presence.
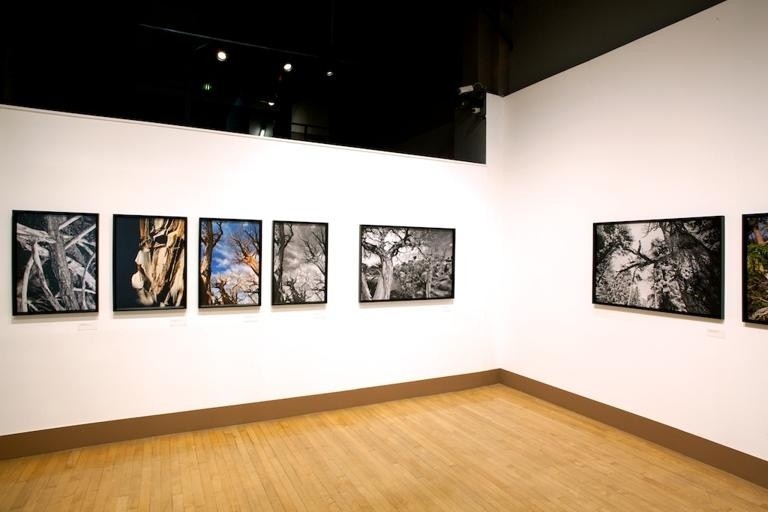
left=472, top=108, right=480, bottom=114
left=457, top=85, right=474, bottom=95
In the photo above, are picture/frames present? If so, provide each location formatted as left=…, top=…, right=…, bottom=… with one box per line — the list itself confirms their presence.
left=198, top=216, right=262, bottom=309
left=593, top=214, right=768, bottom=325
left=11, top=208, right=100, bottom=316
left=359, top=223, right=455, bottom=305
left=271, top=220, right=329, bottom=306
left=112, top=213, right=187, bottom=311
